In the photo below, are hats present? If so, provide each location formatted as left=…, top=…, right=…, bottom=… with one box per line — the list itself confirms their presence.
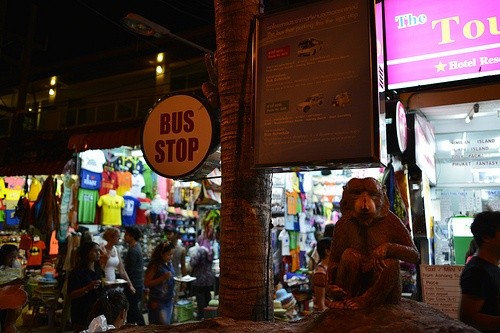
left=163, top=224, right=176, bottom=231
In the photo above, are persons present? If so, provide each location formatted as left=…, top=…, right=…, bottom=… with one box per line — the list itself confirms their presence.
left=144, top=238, right=176, bottom=325
left=311, top=224, right=334, bottom=314
left=161, top=224, right=224, bottom=305
left=0, top=222, right=135, bottom=333
left=400, top=262, right=419, bottom=300
left=96, top=190, right=152, bottom=225
left=459, top=210, right=500, bottom=333
left=122, top=225, right=146, bottom=326
